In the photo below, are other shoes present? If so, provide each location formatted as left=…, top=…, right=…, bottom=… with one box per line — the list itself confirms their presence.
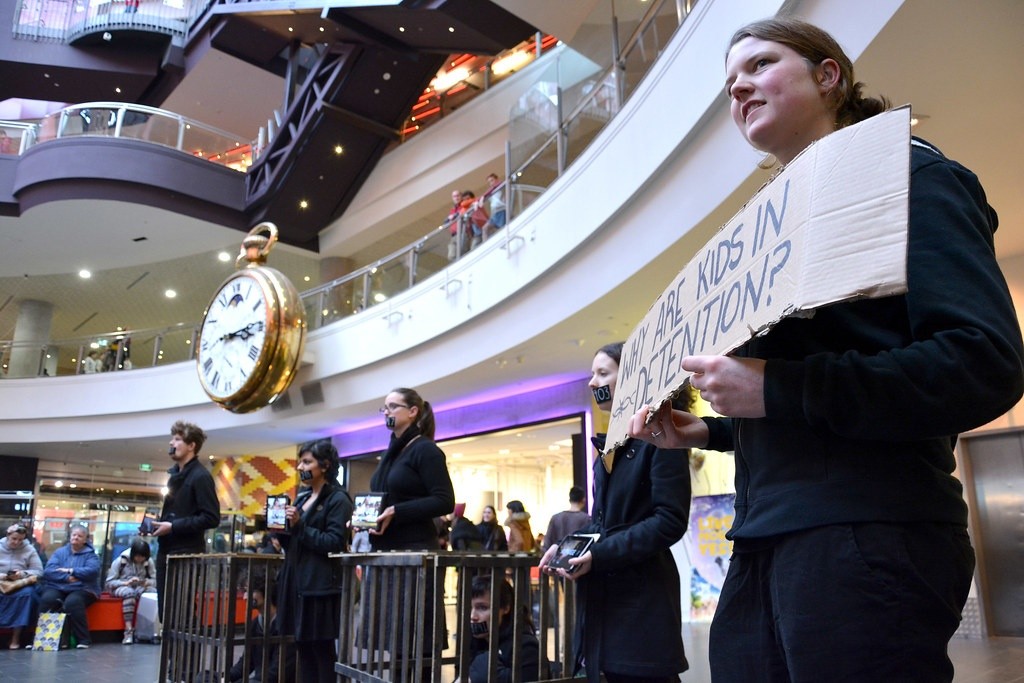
left=10, top=643, right=20, bottom=649
left=122, top=632, right=133, bottom=644
left=77, top=641, right=92, bottom=649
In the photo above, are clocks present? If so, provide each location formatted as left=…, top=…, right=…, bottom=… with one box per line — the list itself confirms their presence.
left=194, top=221, right=307, bottom=415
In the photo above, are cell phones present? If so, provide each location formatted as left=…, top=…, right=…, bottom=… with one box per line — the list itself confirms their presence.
left=6, top=570, right=17, bottom=581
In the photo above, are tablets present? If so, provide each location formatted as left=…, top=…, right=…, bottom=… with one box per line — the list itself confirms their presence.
left=348, top=491, right=386, bottom=531
left=267, top=494, right=289, bottom=531
left=545, top=534, right=594, bottom=572
left=139, top=507, right=160, bottom=533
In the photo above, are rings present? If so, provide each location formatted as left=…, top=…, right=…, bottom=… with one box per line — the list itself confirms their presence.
left=651, top=429, right=663, bottom=438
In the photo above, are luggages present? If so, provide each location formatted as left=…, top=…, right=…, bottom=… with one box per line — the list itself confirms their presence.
left=134, top=593, right=163, bottom=644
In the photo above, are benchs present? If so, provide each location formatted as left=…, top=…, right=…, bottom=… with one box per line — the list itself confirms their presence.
left=0, top=590, right=138, bottom=634
left=195, top=589, right=260, bottom=626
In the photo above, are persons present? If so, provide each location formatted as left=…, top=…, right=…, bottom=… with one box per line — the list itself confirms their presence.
left=263, top=440, right=353, bottom=683
left=82, top=340, right=124, bottom=374
left=197, top=527, right=371, bottom=683
left=352, top=500, right=379, bottom=517
left=268, top=499, right=284, bottom=526
left=0, top=130, right=12, bottom=154
left=345, top=387, right=455, bottom=682
left=538, top=342, right=695, bottom=683
left=628, top=22, right=1024, bottom=683
left=138, top=422, right=220, bottom=683
left=0, top=524, right=102, bottom=650
left=434, top=499, right=536, bottom=614
left=544, top=487, right=590, bottom=549
left=469, top=575, right=551, bottom=683
left=445, top=173, right=506, bottom=261
left=105, top=540, right=159, bottom=644
left=125, top=0, right=139, bottom=13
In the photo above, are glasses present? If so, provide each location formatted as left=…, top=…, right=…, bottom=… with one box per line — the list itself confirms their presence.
left=380, top=402, right=411, bottom=413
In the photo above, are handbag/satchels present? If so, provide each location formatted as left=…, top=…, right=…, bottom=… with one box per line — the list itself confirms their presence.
left=0, top=574, right=37, bottom=593
left=533, top=589, right=560, bottom=631
left=31, top=599, right=70, bottom=651
